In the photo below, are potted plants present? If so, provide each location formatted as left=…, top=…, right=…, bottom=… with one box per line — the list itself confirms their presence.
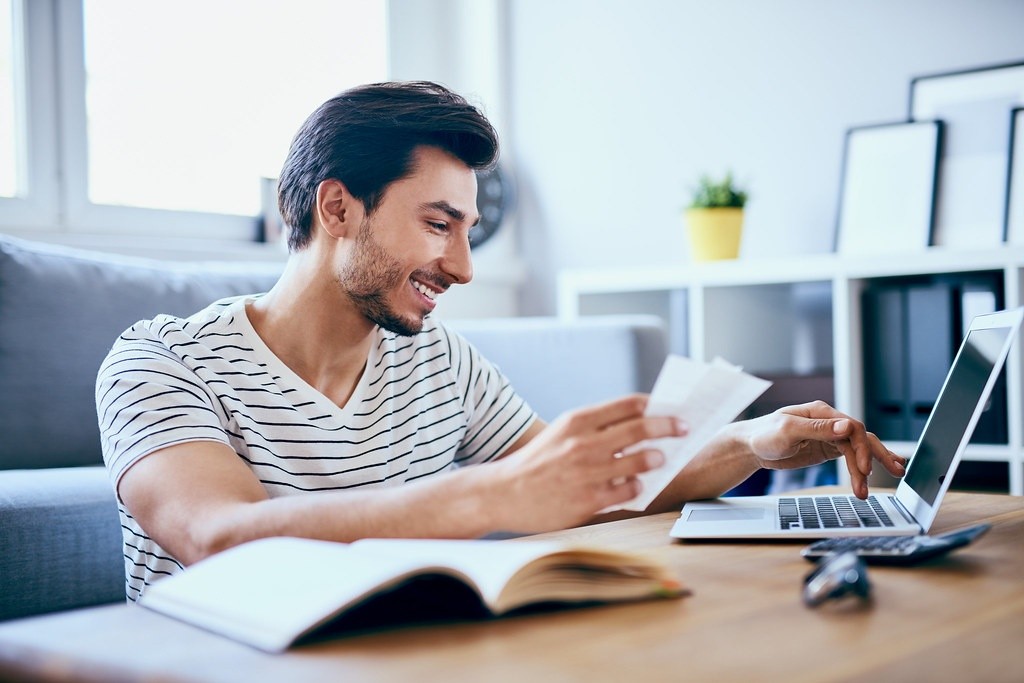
left=684, top=170, right=749, bottom=260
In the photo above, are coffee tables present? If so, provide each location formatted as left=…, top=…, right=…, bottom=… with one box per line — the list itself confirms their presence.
left=0, top=483, right=1024, bottom=683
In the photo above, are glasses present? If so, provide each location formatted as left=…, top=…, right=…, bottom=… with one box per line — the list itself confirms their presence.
left=802, top=553, right=868, bottom=606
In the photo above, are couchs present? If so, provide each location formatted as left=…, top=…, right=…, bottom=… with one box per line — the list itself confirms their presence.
left=0, top=232, right=669, bottom=624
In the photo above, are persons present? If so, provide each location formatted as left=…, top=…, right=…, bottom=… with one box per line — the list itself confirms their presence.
left=94, top=82, right=908, bottom=604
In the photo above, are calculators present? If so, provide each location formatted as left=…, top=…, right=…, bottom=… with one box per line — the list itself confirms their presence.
left=799, top=522, right=992, bottom=566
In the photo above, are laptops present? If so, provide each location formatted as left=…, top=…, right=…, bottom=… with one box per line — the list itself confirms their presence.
left=671, top=306, right=1022, bottom=543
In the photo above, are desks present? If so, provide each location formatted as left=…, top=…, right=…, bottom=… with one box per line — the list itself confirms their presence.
left=554, top=243, right=1024, bottom=497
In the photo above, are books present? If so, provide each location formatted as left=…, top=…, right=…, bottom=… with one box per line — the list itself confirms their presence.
left=139, top=535, right=692, bottom=651
